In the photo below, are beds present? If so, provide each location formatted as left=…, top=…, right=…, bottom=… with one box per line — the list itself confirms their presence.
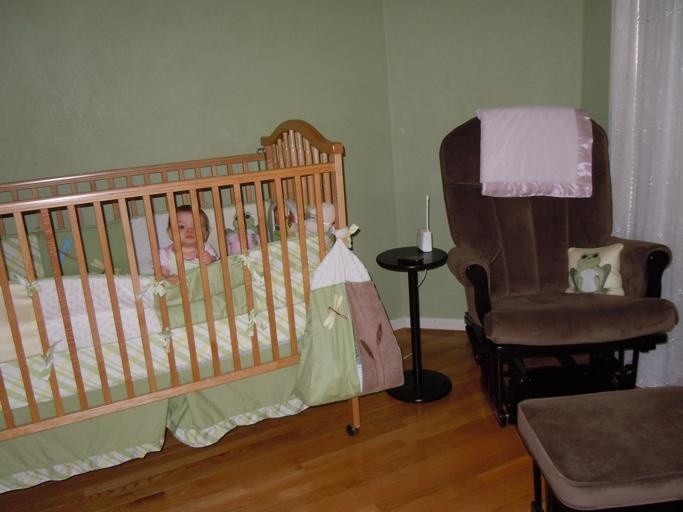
left=0, top=119, right=405, bottom=494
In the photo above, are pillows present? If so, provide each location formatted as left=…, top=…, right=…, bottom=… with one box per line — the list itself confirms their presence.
left=565, top=243, right=626, bottom=298
left=154, top=232, right=334, bottom=330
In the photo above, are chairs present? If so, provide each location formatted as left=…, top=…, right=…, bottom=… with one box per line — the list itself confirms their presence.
left=438, top=108, right=679, bottom=426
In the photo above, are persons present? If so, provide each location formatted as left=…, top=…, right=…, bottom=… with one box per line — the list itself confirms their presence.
left=153, top=203, right=218, bottom=285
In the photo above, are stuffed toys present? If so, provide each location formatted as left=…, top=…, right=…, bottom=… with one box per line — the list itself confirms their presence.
left=224, top=198, right=296, bottom=258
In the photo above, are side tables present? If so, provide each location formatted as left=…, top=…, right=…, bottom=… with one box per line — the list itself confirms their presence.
left=376, top=245, right=452, bottom=403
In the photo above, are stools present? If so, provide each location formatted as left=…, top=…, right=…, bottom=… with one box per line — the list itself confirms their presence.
left=516, top=385, right=683, bottom=510
